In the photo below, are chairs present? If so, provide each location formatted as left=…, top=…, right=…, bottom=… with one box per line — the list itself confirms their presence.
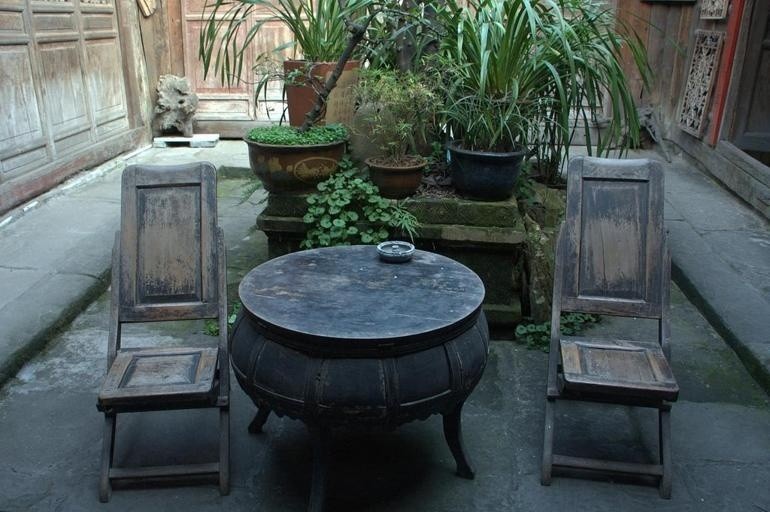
left=540, top=154, right=683, bottom=503
left=92, top=159, right=242, bottom=505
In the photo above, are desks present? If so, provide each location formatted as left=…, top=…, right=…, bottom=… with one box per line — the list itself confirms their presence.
left=230, top=238, right=490, bottom=507
